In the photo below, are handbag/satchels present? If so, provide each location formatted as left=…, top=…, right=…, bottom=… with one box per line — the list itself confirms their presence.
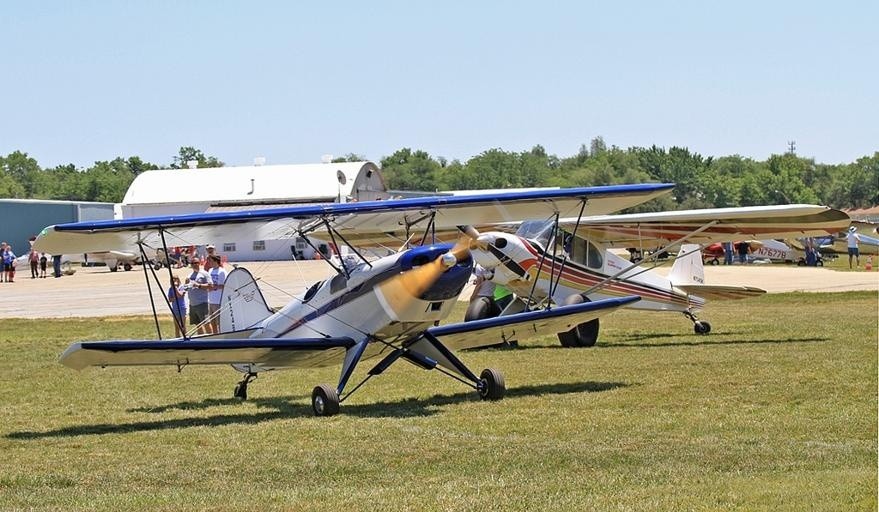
left=12, top=259, right=18, bottom=267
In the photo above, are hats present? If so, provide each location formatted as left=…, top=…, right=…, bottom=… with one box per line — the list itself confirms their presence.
left=206, top=243, right=215, bottom=249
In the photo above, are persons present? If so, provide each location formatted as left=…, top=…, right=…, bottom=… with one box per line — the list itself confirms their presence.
left=721, top=225, right=861, bottom=269
left=470, top=263, right=497, bottom=302
left=0, top=242, right=228, bottom=338
